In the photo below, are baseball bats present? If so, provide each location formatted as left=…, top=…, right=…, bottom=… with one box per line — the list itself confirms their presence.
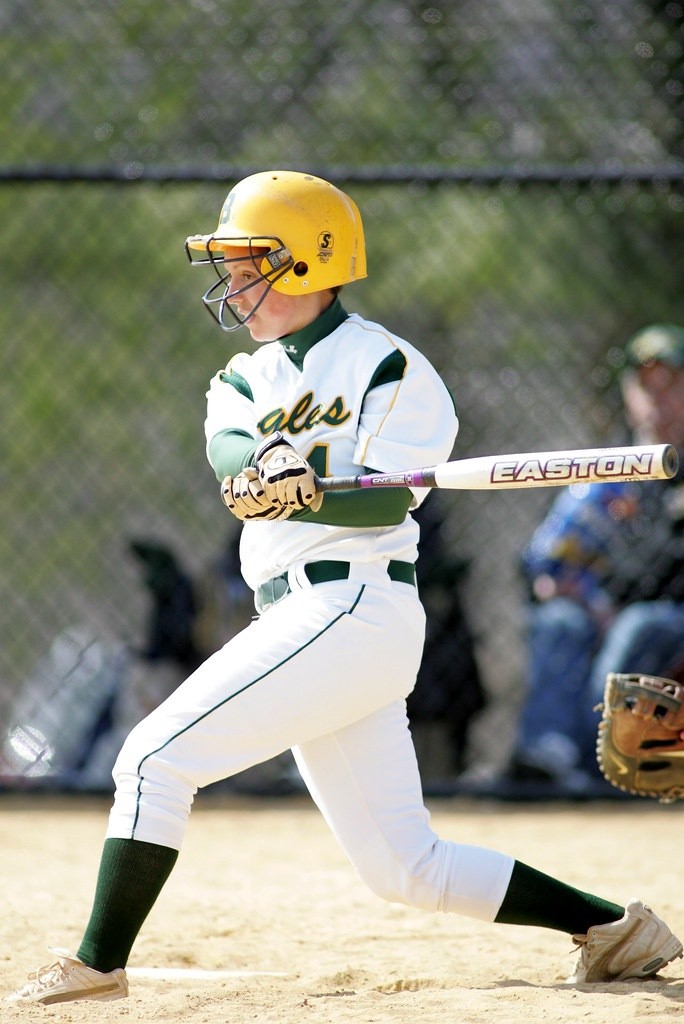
left=219, top=440, right=681, bottom=508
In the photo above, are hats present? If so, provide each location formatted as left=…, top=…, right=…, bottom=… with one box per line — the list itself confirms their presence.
left=617, top=325, right=684, bottom=368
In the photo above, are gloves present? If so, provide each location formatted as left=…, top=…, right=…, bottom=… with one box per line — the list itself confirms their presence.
left=220, top=467, right=292, bottom=524
left=251, top=430, right=326, bottom=513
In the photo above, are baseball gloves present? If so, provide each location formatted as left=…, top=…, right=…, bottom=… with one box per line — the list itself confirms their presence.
left=593, top=671, right=684, bottom=805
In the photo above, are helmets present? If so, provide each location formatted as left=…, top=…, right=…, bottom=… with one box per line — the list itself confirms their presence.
left=182, top=170, right=367, bottom=333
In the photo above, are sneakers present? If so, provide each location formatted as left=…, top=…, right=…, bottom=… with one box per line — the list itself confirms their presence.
left=566, top=899, right=684, bottom=986
left=6, top=952, right=130, bottom=1006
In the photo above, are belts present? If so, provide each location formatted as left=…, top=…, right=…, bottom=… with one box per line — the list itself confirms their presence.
left=256, top=560, right=416, bottom=609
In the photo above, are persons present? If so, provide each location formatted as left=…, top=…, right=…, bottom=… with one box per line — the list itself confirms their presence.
left=10, top=172, right=684, bottom=1005
left=492, top=324, right=684, bottom=784
